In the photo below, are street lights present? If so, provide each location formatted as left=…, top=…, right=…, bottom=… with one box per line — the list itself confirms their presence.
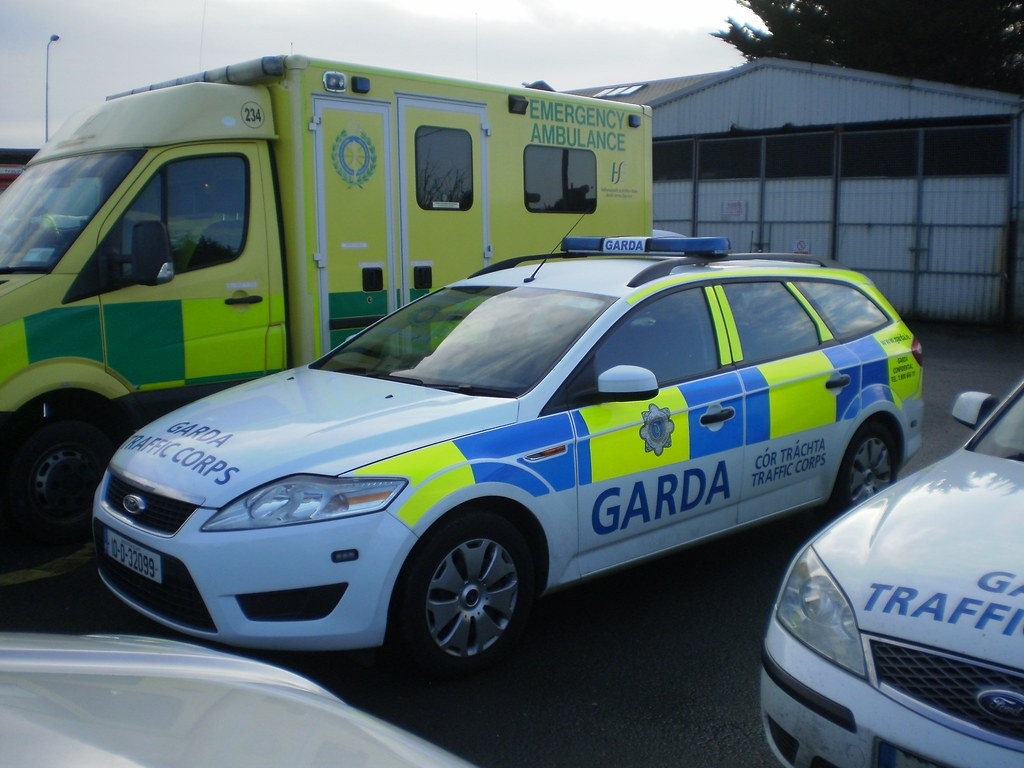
left=46, top=34, right=59, bottom=143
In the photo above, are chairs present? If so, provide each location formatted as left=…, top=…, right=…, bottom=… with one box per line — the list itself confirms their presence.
left=185, top=188, right=246, bottom=272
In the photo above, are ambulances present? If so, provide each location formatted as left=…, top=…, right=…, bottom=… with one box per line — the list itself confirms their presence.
left=1, top=56, right=654, bottom=542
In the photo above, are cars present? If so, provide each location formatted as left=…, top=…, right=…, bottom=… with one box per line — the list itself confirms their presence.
left=1, top=633, right=479, bottom=768
left=759, top=379, right=1024, bottom=768
left=92, top=237, right=924, bottom=676
